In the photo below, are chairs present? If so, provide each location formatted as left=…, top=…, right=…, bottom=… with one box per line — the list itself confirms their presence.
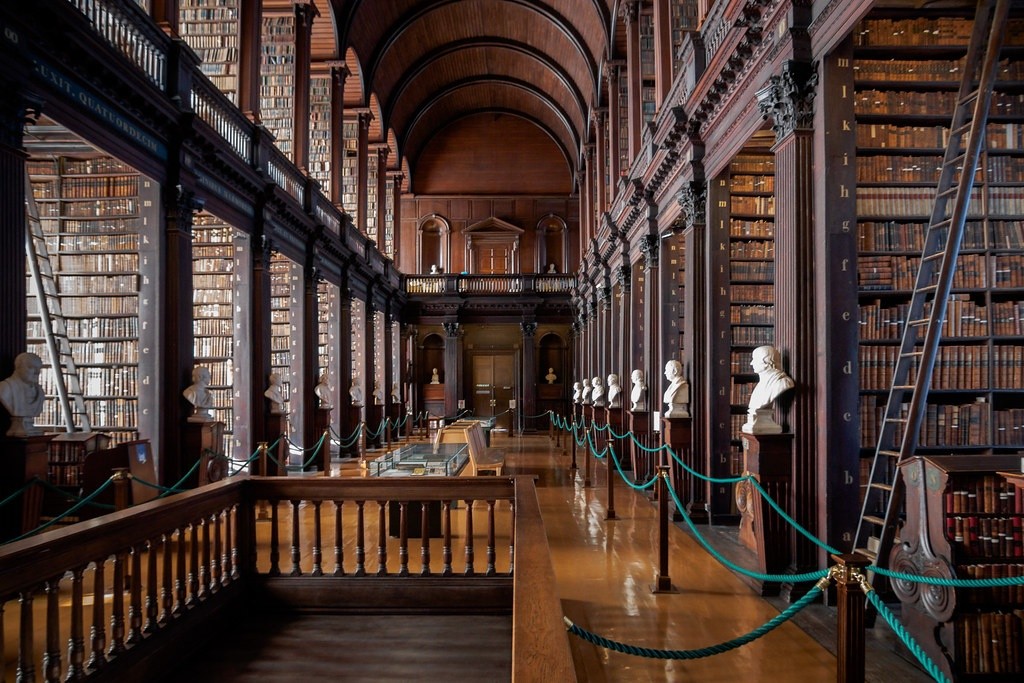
left=465, top=421, right=507, bottom=477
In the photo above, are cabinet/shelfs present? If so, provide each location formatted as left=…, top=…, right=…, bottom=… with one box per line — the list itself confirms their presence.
left=26, top=0, right=398, bottom=499
left=675, top=1, right=1024, bottom=683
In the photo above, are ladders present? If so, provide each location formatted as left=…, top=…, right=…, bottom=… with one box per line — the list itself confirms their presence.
left=24, top=164, right=94, bottom=434
left=853, top=0, right=1012, bottom=630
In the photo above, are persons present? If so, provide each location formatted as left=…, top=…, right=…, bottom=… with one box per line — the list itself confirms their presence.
left=582, top=379, right=592, bottom=399
left=373, top=381, right=383, bottom=400
left=607, top=374, right=621, bottom=402
left=591, top=377, right=604, bottom=401
left=431, top=368, right=438, bottom=382
left=0, top=353, right=46, bottom=417
left=631, top=369, right=647, bottom=402
left=183, top=366, right=213, bottom=408
left=572, top=382, right=582, bottom=399
left=315, top=374, right=331, bottom=403
left=545, top=368, right=556, bottom=381
left=663, top=360, right=689, bottom=403
left=349, top=377, right=362, bottom=401
left=264, top=373, right=283, bottom=403
left=746, top=345, right=794, bottom=410
left=392, top=383, right=399, bottom=401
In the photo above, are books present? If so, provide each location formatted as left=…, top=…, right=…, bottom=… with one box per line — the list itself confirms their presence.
left=0, top=1, right=1024, bottom=673
